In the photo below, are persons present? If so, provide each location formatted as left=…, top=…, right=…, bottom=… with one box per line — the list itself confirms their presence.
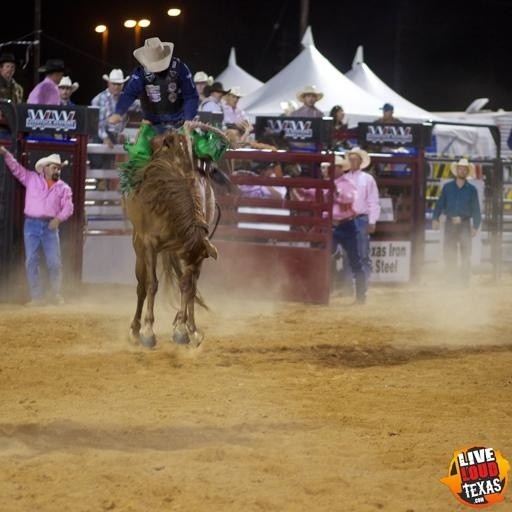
left=296, top=152, right=366, bottom=304
left=2, top=58, right=407, bottom=233
left=430, top=158, right=482, bottom=290
left=108, top=38, right=230, bottom=184
left=335, top=148, right=380, bottom=300
left=0, top=145, right=75, bottom=306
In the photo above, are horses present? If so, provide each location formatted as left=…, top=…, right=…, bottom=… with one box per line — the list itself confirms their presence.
left=118, top=127, right=218, bottom=351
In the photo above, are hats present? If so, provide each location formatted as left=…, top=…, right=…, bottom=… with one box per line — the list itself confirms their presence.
left=193, top=70, right=245, bottom=97
left=379, top=103, right=394, bottom=110
left=280, top=100, right=299, bottom=116
left=35, top=154, right=69, bottom=174
left=38, top=58, right=74, bottom=72
left=133, top=37, right=175, bottom=72
left=102, top=68, right=131, bottom=84
left=344, top=146, right=371, bottom=169
left=295, top=85, right=324, bottom=103
left=449, top=159, right=475, bottom=177
left=58, top=76, right=79, bottom=94
left=321, top=155, right=352, bottom=171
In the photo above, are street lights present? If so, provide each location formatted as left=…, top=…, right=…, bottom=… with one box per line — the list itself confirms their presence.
left=123, top=19, right=151, bottom=51
left=93, top=24, right=109, bottom=50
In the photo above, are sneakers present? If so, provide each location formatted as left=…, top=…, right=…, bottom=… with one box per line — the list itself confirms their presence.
left=24, top=299, right=47, bottom=306
left=51, top=297, right=65, bottom=306
